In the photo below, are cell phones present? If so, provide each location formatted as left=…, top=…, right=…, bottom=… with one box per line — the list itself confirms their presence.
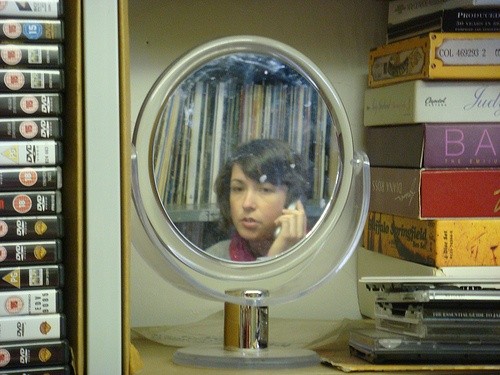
left=276, top=195, right=306, bottom=237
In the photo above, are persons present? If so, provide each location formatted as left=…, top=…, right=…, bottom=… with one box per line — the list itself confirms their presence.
left=197, top=139, right=311, bottom=262
